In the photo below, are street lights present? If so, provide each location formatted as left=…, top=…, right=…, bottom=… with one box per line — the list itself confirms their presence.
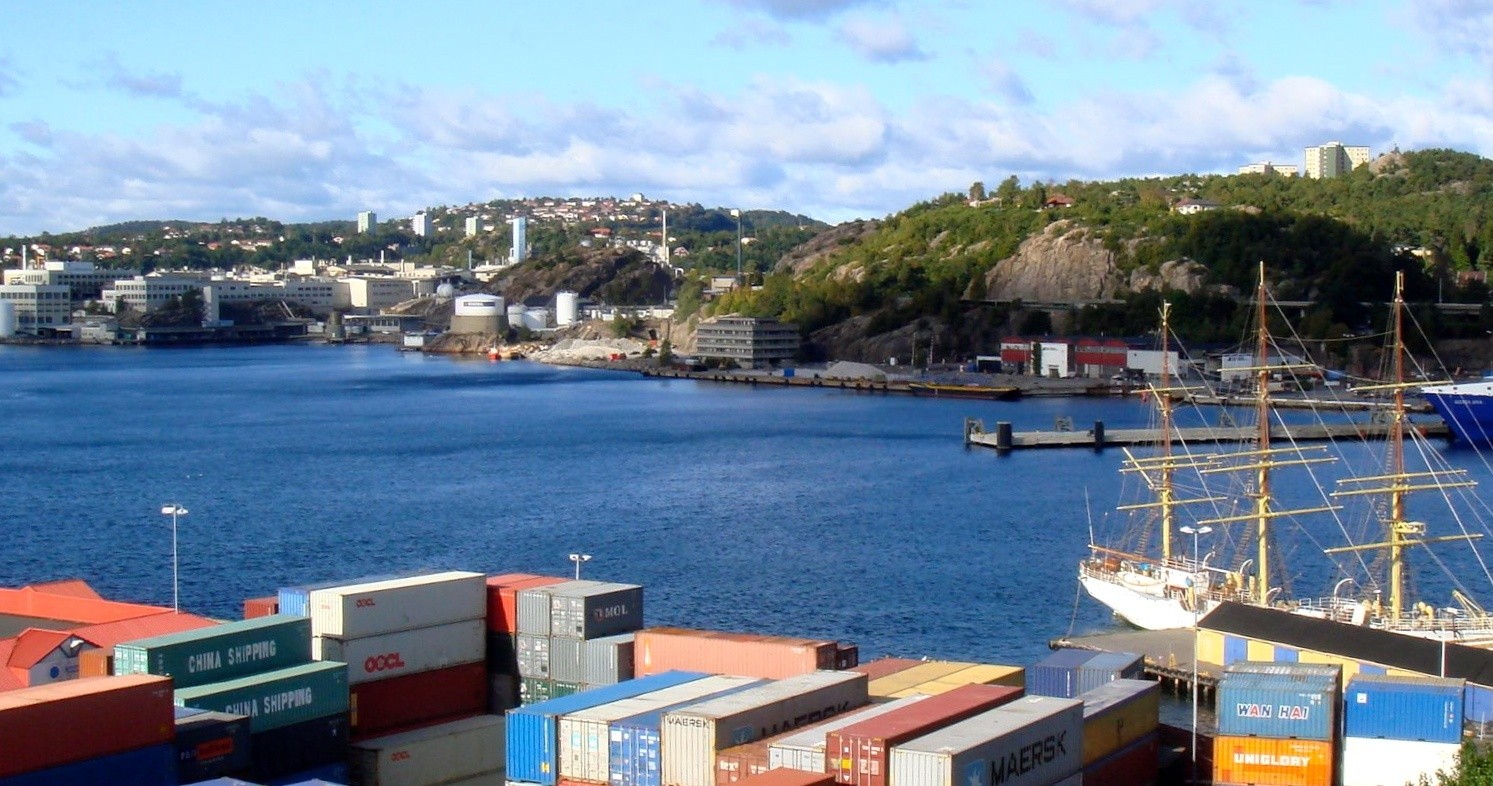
left=161, top=502, right=189, bottom=614
left=569, top=552, right=592, bottom=581
left=729, top=208, right=743, bottom=275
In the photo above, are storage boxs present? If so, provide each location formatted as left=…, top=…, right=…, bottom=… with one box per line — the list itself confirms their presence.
left=0, top=569, right=1467, bottom=786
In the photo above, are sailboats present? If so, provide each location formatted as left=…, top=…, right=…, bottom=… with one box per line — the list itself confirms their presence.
left=1077, top=261, right=1493, bottom=655
